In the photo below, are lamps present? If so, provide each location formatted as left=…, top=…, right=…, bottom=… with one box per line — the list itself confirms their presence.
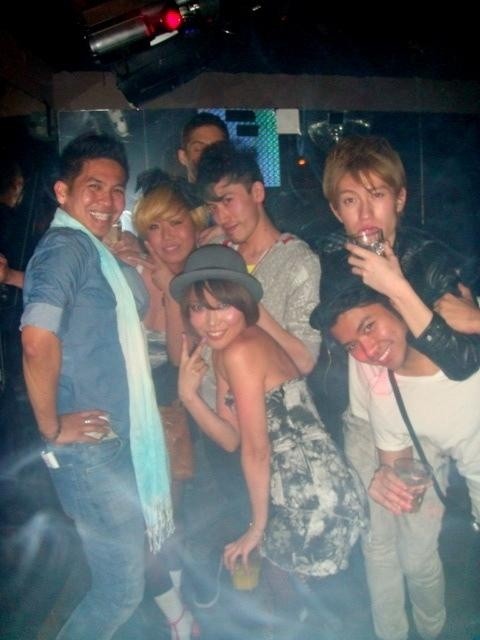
left=80, top=0, right=261, bottom=108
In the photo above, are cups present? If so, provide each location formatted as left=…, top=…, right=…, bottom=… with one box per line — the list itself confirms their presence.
left=346, top=227, right=387, bottom=261
left=111, top=220, right=123, bottom=247
left=229, top=548, right=262, bottom=590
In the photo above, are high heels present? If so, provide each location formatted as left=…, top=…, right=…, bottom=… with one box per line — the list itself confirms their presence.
left=167, top=608, right=202, bottom=640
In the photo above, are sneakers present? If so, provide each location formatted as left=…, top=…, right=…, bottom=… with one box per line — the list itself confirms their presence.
left=192, top=552, right=222, bottom=608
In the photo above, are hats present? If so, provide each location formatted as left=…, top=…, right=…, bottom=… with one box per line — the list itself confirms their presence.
left=165, top=244, right=264, bottom=308
left=308, top=260, right=392, bottom=332
